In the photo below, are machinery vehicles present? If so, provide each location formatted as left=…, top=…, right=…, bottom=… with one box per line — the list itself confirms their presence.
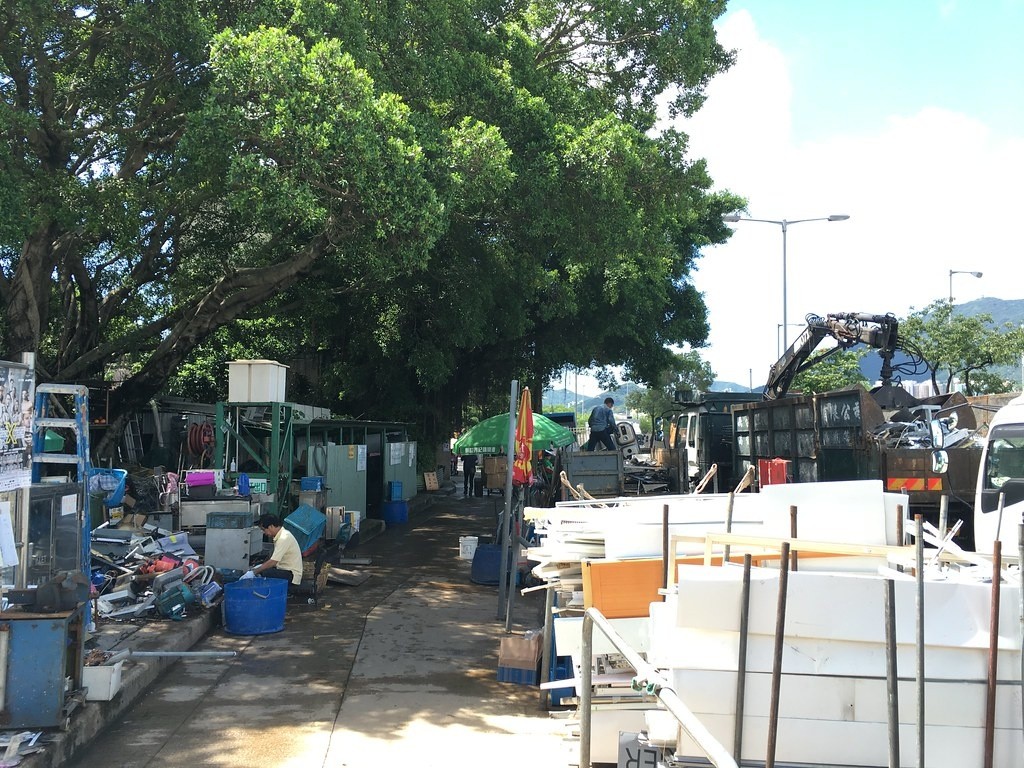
left=648, top=309, right=981, bottom=496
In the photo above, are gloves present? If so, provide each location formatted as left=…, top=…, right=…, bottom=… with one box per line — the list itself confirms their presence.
left=239, top=571, right=256, bottom=581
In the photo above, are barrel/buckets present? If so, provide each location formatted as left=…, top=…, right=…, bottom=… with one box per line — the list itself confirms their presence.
left=380, top=500, right=409, bottom=527
left=225, top=577, right=288, bottom=635
left=457, top=535, right=477, bottom=557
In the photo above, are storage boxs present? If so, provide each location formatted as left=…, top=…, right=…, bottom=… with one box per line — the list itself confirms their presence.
left=87, top=467, right=128, bottom=508
left=484, top=456, right=508, bottom=490
left=81, top=649, right=124, bottom=702
left=285, top=503, right=328, bottom=554
left=495, top=631, right=545, bottom=686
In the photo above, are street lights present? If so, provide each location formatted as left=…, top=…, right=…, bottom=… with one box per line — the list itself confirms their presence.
left=580, top=399, right=583, bottom=419
left=721, top=214, right=851, bottom=354
left=948, top=269, right=982, bottom=329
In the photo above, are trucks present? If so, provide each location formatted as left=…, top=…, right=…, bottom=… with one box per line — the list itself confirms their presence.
left=972, top=390, right=1024, bottom=562
left=654, top=387, right=984, bottom=529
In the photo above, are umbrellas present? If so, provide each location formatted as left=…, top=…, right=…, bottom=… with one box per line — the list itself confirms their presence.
left=510, top=385, right=535, bottom=551
left=453, top=412, right=576, bottom=502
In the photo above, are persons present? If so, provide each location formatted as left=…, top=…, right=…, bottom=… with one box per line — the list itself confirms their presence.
left=239, top=514, right=303, bottom=589
left=452, top=453, right=458, bottom=475
left=587, top=397, right=619, bottom=452
left=460, top=455, right=478, bottom=497
left=542, top=448, right=555, bottom=486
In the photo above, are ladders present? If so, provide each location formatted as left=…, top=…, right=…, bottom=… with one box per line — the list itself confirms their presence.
left=124, top=413, right=145, bottom=463
left=34, top=382, right=95, bottom=634
